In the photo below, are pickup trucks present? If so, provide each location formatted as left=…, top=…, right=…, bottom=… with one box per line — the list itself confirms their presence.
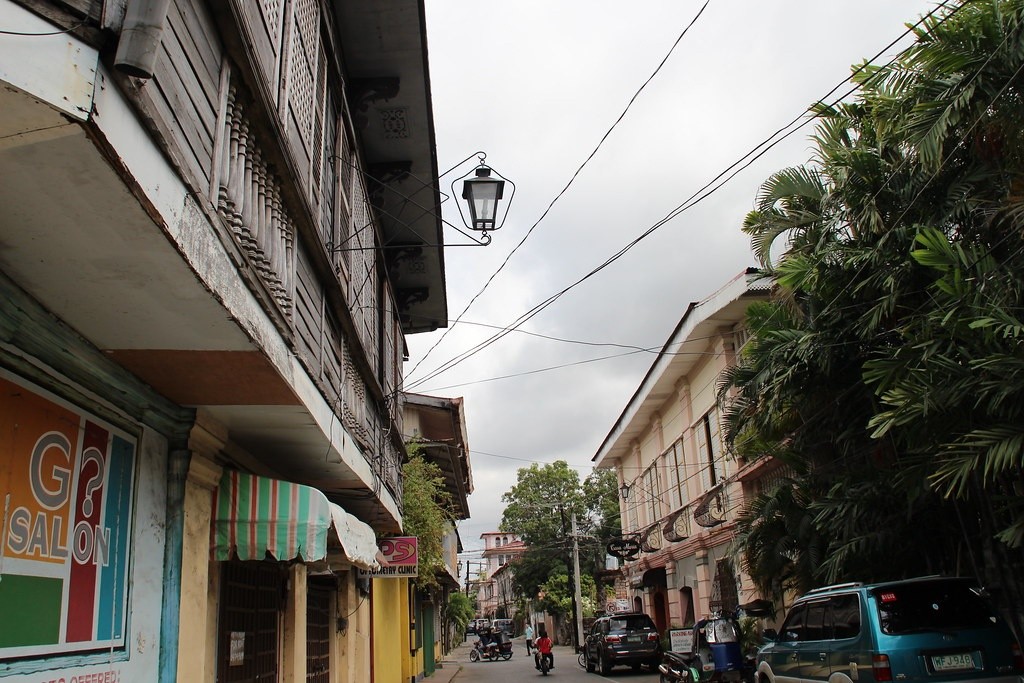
left=467, top=618, right=514, bottom=639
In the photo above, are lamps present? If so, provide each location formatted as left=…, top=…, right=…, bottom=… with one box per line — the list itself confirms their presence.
left=621, top=482, right=664, bottom=503
left=327, top=151, right=515, bottom=252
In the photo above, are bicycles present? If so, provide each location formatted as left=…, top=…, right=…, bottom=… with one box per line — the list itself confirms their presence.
left=577, top=646, right=586, bottom=667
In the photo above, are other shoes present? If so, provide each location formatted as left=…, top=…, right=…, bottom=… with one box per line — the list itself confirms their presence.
left=549, top=666, right=555, bottom=668
left=535, top=666, right=539, bottom=668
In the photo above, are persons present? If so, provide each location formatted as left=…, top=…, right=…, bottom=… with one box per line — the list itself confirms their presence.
left=532, top=629, right=555, bottom=670
left=525, top=623, right=534, bottom=656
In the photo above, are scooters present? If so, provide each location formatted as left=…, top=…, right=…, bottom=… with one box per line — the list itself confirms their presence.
left=540, top=654, right=549, bottom=676
left=469, top=641, right=513, bottom=662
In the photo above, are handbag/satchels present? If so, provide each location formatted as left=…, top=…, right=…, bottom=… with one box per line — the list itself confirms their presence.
left=537, top=651, right=542, bottom=659
left=532, top=648, right=537, bottom=654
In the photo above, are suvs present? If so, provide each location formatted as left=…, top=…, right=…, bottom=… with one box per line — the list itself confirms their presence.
left=584, top=611, right=664, bottom=675
left=754, top=574, right=1024, bottom=682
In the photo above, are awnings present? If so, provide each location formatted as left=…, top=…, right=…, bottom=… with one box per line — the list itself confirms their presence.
left=209, top=470, right=381, bottom=573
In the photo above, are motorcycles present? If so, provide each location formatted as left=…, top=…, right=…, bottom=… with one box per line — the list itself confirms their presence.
left=658, top=650, right=691, bottom=683
